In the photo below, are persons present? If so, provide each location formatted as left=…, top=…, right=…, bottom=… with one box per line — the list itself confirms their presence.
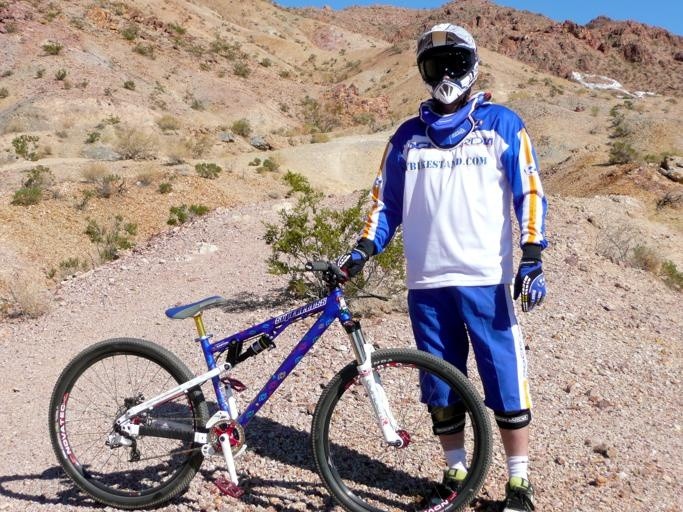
left=323, top=23, right=547, bottom=512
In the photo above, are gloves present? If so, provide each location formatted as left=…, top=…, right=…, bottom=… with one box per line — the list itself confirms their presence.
left=321, top=236, right=377, bottom=285
left=513, top=254, right=547, bottom=314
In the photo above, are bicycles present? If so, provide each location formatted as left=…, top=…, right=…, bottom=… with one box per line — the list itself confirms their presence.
left=48, top=263, right=493, bottom=506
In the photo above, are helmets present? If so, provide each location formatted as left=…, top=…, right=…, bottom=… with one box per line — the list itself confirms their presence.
left=415, top=22, right=480, bottom=106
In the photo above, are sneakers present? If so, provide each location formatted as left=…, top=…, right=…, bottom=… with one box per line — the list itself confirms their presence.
left=501, top=474, right=536, bottom=512
left=404, top=464, right=475, bottom=512
